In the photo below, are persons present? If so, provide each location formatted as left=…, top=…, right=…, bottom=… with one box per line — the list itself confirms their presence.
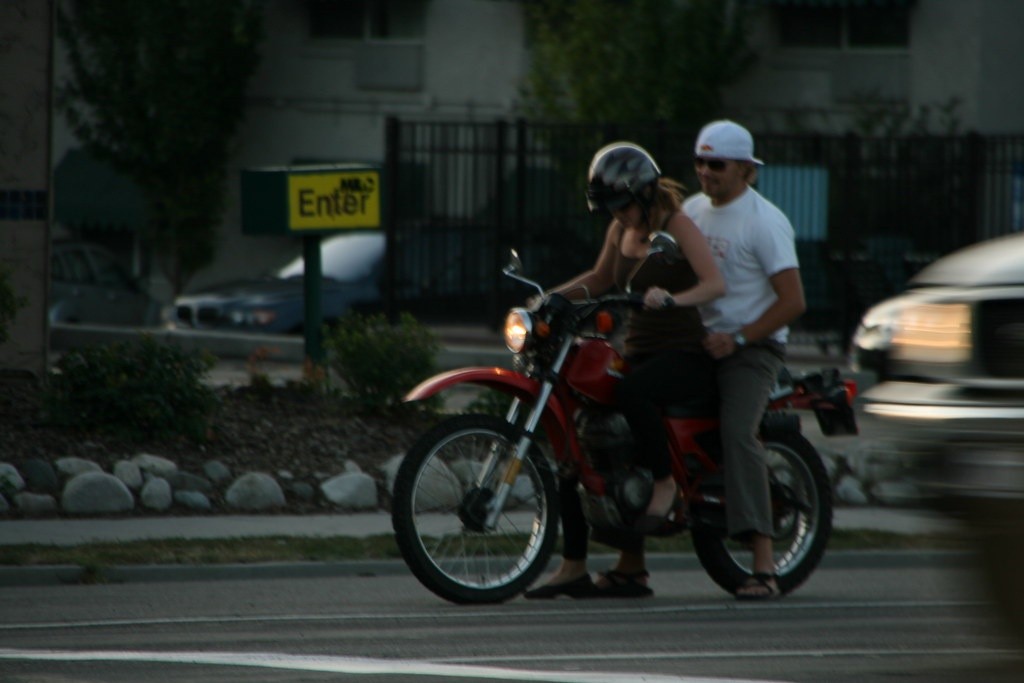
left=587, top=117, right=806, bottom=603
left=521, top=139, right=729, bottom=599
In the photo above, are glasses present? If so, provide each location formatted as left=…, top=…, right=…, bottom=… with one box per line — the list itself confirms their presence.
left=693, top=157, right=744, bottom=171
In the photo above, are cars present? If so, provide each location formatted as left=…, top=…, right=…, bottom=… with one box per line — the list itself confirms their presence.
left=851, top=233, right=1024, bottom=505
left=173, top=233, right=391, bottom=336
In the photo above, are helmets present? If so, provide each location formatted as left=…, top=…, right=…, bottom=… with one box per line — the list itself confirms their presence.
left=586, top=141, right=662, bottom=212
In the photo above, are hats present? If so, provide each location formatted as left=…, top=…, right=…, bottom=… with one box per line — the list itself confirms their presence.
left=695, top=119, right=763, bottom=164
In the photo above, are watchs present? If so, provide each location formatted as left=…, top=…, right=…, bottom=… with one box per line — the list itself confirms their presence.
left=732, top=329, right=752, bottom=352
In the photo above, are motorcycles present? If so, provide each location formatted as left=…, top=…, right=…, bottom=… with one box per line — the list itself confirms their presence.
left=390, top=246, right=857, bottom=605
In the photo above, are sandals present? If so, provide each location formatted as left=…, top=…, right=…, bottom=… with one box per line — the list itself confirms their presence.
left=737, top=571, right=782, bottom=599
left=592, top=566, right=654, bottom=598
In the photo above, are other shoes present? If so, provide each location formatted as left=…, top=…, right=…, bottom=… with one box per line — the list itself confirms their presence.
left=524, top=572, right=595, bottom=599
left=645, top=483, right=683, bottom=531
